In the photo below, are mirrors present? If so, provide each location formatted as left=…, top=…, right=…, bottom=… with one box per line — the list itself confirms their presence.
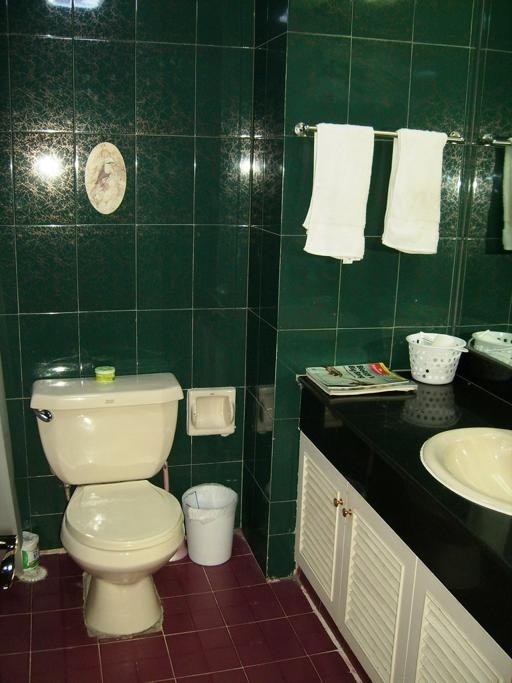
left=457, top=0, right=512, bottom=370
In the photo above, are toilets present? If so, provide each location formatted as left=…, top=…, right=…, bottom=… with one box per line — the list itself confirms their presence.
left=33, top=373, right=186, bottom=634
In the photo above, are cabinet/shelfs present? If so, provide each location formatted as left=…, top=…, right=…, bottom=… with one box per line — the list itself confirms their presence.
left=295, top=426, right=511, bottom=683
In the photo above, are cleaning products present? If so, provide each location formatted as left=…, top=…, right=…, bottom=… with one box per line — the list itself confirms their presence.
left=15, top=531, right=38, bottom=571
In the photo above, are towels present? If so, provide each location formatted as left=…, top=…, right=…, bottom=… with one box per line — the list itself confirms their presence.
left=501, top=136, right=511, bottom=249
left=301, top=121, right=448, bottom=265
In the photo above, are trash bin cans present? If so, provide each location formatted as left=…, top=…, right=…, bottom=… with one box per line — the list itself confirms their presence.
left=181, top=484, right=237, bottom=566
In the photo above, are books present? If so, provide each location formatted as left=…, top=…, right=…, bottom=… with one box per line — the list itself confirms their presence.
left=305, top=362, right=418, bottom=396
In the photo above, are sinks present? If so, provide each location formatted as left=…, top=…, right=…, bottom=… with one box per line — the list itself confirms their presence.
left=420, top=427, right=512, bottom=517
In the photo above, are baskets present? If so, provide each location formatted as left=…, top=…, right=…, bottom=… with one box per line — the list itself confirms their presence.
left=399, top=382, right=457, bottom=428
left=405, top=332, right=467, bottom=387
left=472, top=331, right=512, bottom=359
